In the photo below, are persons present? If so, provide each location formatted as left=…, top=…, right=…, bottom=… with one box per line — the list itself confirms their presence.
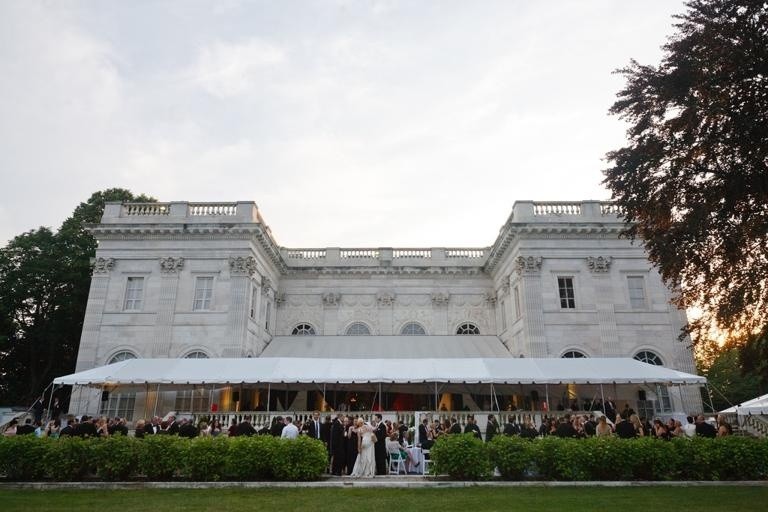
left=300, top=411, right=482, bottom=478
left=485, top=412, right=733, bottom=444
left=60, top=413, right=302, bottom=442
left=6, top=397, right=62, bottom=440
left=210, top=396, right=634, bottom=424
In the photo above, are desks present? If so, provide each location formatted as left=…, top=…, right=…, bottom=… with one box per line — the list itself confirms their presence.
left=391, top=446, right=423, bottom=473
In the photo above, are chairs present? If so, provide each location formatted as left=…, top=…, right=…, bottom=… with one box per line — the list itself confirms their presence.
left=421, top=449, right=437, bottom=476
left=388, top=449, right=407, bottom=474
